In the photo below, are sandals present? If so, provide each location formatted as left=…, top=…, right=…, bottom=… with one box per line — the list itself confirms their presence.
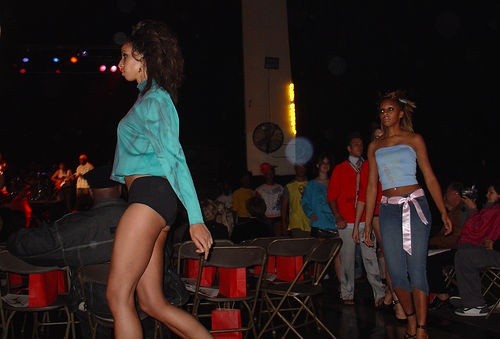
left=415, top=325, right=428, bottom=339
left=404, top=312, right=417, bottom=339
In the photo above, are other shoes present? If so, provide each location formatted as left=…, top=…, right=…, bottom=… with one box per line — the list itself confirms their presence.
left=392, top=300, right=407, bottom=326
left=344, top=300, right=355, bottom=305
left=378, top=298, right=384, bottom=306
left=428, top=296, right=449, bottom=311
left=380, top=301, right=392, bottom=314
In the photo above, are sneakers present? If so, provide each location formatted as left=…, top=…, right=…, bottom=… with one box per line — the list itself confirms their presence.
left=454, top=304, right=488, bottom=316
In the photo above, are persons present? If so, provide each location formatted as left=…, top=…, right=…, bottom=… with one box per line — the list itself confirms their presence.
left=189, top=136, right=500, bottom=323
left=363, top=90, right=453, bottom=339
left=50, top=162, right=73, bottom=200
left=71, top=153, right=95, bottom=204
left=105, top=23, right=213, bottom=339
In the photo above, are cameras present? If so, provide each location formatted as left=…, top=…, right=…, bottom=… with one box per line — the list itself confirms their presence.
left=462, top=185, right=480, bottom=198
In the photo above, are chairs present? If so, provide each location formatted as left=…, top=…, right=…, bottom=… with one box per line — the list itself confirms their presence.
left=0, top=183, right=500, bottom=339
